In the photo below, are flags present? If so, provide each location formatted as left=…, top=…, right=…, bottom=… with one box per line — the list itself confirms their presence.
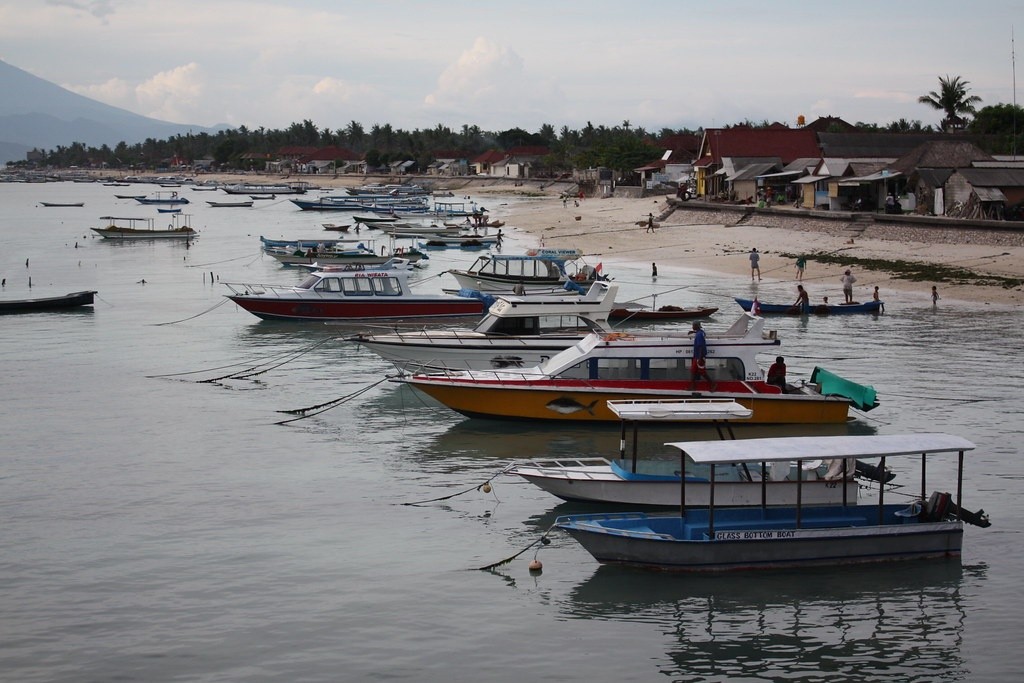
left=595, top=262, right=602, bottom=272
left=751, top=297, right=759, bottom=315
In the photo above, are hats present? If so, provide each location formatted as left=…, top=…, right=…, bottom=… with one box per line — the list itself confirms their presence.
left=694, top=321, right=702, bottom=329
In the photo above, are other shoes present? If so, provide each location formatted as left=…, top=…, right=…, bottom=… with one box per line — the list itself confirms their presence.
left=711, top=383, right=717, bottom=392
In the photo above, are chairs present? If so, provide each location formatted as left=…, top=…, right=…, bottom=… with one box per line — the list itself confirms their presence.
left=894, top=504, right=922, bottom=525
left=801, top=459, right=823, bottom=481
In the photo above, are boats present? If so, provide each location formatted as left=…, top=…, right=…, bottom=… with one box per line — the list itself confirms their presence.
left=352, top=216, right=399, bottom=223
left=472, top=221, right=506, bottom=228
left=500, top=396, right=861, bottom=513
left=322, top=224, right=351, bottom=231
left=421, top=234, right=500, bottom=244
left=0, top=290, right=99, bottom=312
left=325, top=279, right=733, bottom=374
left=388, top=232, right=435, bottom=238
left=733, top=295, right=885, bottom=318
left=375, top=223, right=463, bottom=234
left=444, top=223, right=471, bottom=230
left=365, top=221, right=420, bottom=229
left=553, top=434, right=978, bottom=569
left=386, top=296, right=857, bottom=427
left=24, top=175, right=484, bottom=218
left=448, top=248, right=608, bottom=293
left=89, top=217, right=198, bottom=240
left=218, top=257, right=483, bottom=321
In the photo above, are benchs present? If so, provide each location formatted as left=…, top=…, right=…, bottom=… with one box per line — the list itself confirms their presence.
left=787, top=382, right=820, bottom=396
left=842, top=202, right=863, bottom=211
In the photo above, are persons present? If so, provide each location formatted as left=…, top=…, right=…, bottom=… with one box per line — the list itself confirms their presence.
left=839, top=269, right=856, bottom=302
left=512, top=280, right=526, bottom=296
left=886, top=192, right=895, bottom=209
left=766, top=187, right=772, bottom=207
left=354, top=223, right=360, bottom=234
left=564, top=273, right=584, bottom=294
left=749, top=248, right=762, bottom=280
left=793, top=285, right=809, bottom=313
left=496, top=229, right=503, bottom=248
left=767, top=356, right=787, bottom=390
left=687, top=321, right=717, bottom=392
left=873, top=285, right=884, bottom=311
left=651, top=262, right=657, bottom=277
left=794, top=252, right=806, bottom=280
left=823, top=296, right=828, bottom=304
left=562, top=194, right=568, bottom=209
left=646, top=213, right=655, bottom=233
left=931, top=285, right=939, bottom=304
left=573, top=200, right=580, bottom=207
left=578, top=190, right=584, bottom=200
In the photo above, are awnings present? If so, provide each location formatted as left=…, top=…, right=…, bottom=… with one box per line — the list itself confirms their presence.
left=755, top=171, right=902, bottom=183
left=633, top=167, right=659, bottom=171
